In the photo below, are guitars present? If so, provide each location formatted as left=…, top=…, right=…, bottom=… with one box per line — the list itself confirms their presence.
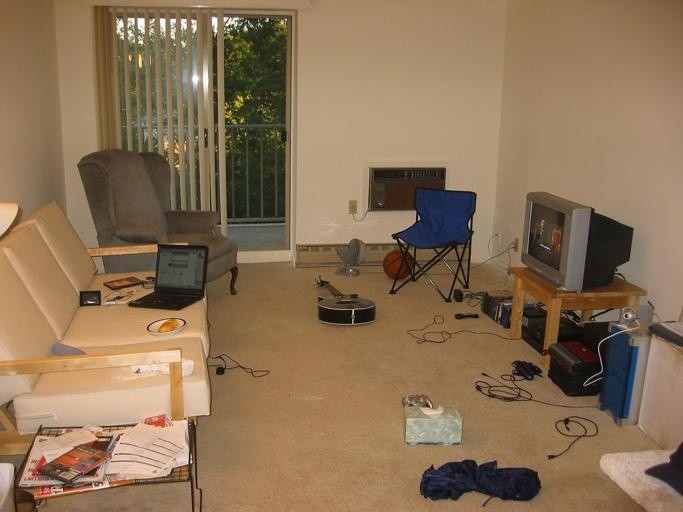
left=313, top=274, right=375, bottom=326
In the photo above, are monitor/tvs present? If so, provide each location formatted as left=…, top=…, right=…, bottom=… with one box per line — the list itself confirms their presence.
left=521, top=192, right=633, bottom=293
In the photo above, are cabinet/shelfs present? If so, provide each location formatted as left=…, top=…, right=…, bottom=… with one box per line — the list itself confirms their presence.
left=598, top=322, right=652, bottom=426
left=637, top=320, right=682, bottom=450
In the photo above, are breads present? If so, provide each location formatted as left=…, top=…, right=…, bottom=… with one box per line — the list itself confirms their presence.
left=158, top=318, right=179, bottom=333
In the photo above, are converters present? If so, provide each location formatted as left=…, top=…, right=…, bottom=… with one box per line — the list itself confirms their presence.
left=453, top=289, right=463, bottom=303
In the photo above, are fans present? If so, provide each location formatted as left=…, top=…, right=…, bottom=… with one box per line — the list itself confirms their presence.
left=334, top=239, right=367, bottom=278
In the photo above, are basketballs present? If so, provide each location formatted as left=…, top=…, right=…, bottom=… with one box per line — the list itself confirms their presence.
left=383, top=250, right=413, bottom=279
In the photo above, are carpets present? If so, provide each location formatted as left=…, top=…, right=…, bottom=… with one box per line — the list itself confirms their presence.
left=600, top=450, right=682, bottom=512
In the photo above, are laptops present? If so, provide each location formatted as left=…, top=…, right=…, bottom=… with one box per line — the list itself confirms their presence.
left=128, top=244, right=208, bottom=311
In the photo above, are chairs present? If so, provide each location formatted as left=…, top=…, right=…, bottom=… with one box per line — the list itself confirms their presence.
left=388, top=186, right=477, bottom=304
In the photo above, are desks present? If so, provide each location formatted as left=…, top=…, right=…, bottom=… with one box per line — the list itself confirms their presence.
left=14, top=420, right=202, bottom=512
left=506, top=266, right=647, bottom=367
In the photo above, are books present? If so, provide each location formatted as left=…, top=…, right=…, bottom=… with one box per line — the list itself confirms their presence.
left=17, top=414, right=192, bottom=500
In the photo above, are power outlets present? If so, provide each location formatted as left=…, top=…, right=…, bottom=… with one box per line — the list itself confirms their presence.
left=348, top=200, right=357, bottom=215
left=513, top=237, right=520, bottom=254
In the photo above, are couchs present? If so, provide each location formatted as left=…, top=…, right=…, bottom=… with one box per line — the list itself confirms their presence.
left=76, top=150, right=238, bottom=296
left=1, top=199, right=210, bottom=456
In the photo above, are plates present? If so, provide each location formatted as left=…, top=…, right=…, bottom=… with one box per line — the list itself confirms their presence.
left=146, top=317, right=186, bottom=336
